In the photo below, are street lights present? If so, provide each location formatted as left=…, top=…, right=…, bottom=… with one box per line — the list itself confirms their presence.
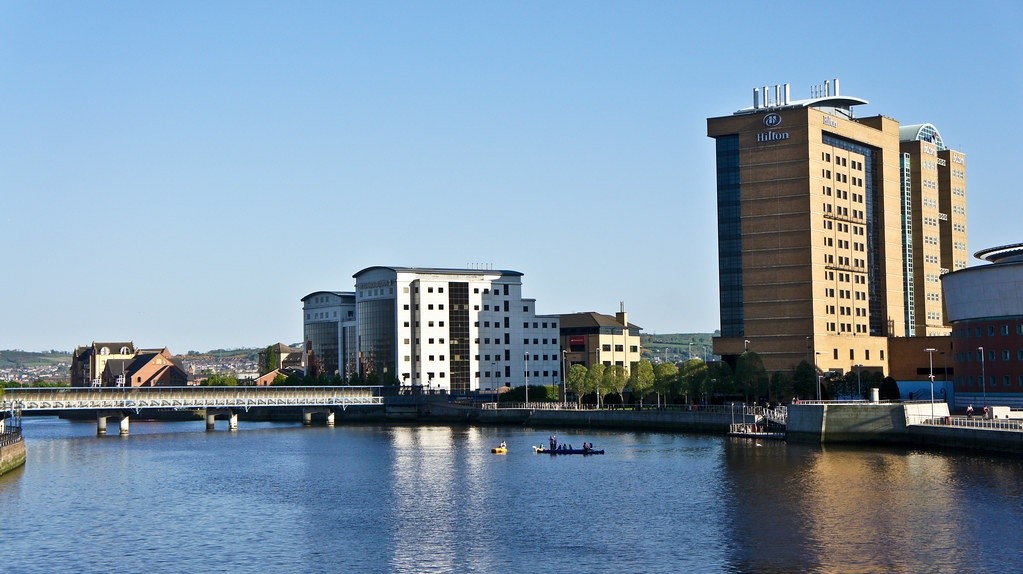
left=524, top=352, right=530, bottom=407
left=702, top=346, right=707, bottom=412
left=744, top=340, right=750, bottom=405
left=638, top=346, right=643, bottom=407
left=562, top=350, right=567, bottom=408
left=924, top=348, right=938, bottom=425
left=664, top=348, right=669, bottom=408
left=979, top=347, right=986, bottom=425
left=688, top=342, right=693, bottom=411
left=596, top=348, right=601, bottom=409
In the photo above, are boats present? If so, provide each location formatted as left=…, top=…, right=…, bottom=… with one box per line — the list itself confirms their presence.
left=490, top=447, right=508, bottom=454
left=538, top=449, right=605, bottom=455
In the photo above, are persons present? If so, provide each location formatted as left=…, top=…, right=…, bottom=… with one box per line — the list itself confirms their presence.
left=540, top=443, right=544, bottom=448
left=982, top=405, right=990, bottom=420
left=499, top=441, right=506, bottom=449
left=569, top=444, right=573, bottom=450
left=563, top=444, right=568, bottom=450
left=583, top=442, right=594, bottom=450
left=966, top=404, right=974, bottom=416
left=558, top=445, right=561, bottom=450
